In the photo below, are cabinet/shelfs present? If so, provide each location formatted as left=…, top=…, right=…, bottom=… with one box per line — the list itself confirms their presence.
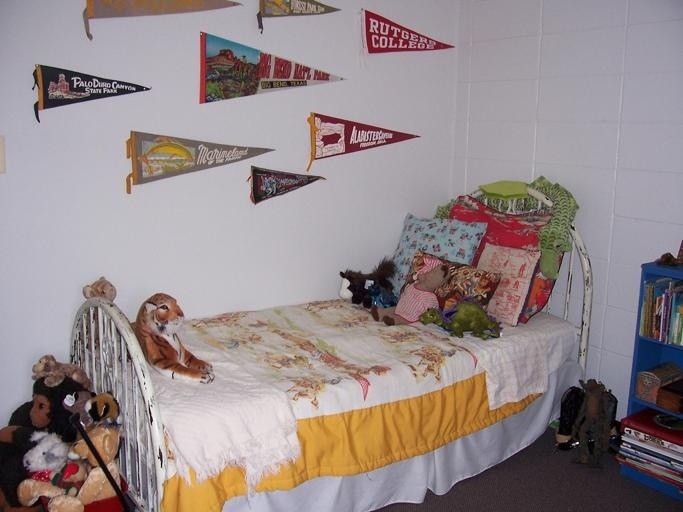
left=618, top=257, right=682, bottom=501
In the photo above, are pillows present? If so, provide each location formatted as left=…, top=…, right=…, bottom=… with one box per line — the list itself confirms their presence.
left=387, top=194, right=568, bottom=332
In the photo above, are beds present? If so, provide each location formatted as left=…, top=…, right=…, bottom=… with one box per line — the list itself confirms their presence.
left=71, top=182, right=592, bottom=510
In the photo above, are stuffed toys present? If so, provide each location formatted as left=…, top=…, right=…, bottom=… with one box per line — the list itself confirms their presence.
left=126, top=292, right=215, bottom=386
left=336, top=252, right=505, bottom=340
left=2, top=353, right=135, bottom=512
left=542, top=376, right=624, bottom=468
left=80, top=274, right=119, bottom=305
left=429, top=177, right=581, bottom=283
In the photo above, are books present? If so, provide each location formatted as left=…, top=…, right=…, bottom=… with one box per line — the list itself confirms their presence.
left=638, top=275, right=683, bottom=348
left=615, top=407, right=681, bottom=494
